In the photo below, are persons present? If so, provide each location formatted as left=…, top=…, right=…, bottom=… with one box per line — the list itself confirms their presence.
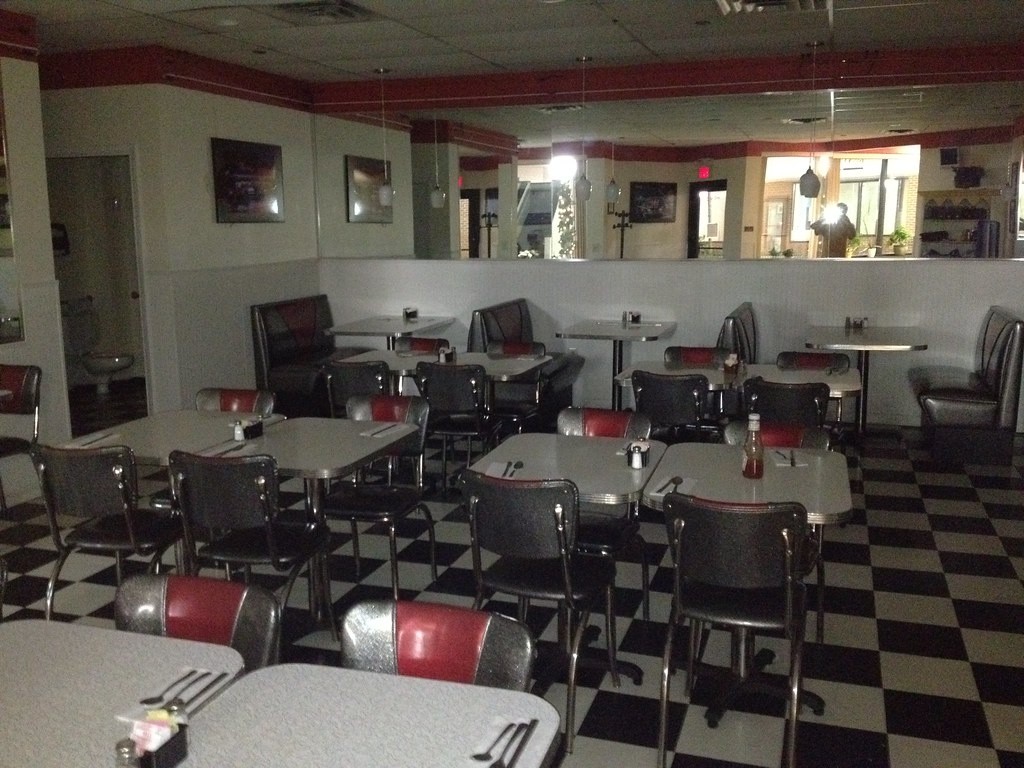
left=811, top=203, right=856, bottom=259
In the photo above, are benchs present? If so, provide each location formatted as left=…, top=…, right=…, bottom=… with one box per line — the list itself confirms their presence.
left=250, top=294, right=376, bottom=418
left=447, top=299, right=585, bottom=430
left=906, top=305, right=1024, bottom=466
left=662, top=304, right=759, bottom=371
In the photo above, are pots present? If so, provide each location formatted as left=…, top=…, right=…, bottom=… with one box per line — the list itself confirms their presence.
left=955, top=166, right=980, bottom=188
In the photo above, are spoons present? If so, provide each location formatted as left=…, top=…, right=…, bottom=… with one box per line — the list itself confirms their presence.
left=472, top=719, right=537, bottom=768
left=140, top=669, right=228, bottom=712
left=673, top=476, right=683, bottom=493
left=508, top=461, right=524, bottom=478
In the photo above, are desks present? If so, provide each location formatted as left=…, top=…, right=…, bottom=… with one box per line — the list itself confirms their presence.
left=0, top=312, right=931, bottom=768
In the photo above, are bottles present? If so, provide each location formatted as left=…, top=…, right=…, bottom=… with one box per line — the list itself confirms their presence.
left=631, top=446, right=643, bottom=469
left=116, top=740, right=140, bottom=768
left=234, top=421, right=245, bottom=441
left=845, top=316, right=869, bottom=328
left=740, top=413, right=765, bottom=479
left=403, top=308, right=411, bottom=319
left=638, top=437, right=646, bottom=442
left=257, top=414, right=266, bottom=433
left=451, top=347, right=456, bottom=360
left=168, top=698, right=191, bottom=744
left=440, top=349, right=446, bottom=363
left=622, top=311, right=632, bottom=322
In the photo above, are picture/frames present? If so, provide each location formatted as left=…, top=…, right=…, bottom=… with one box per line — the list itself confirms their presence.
left=630, top=181, right=676, bottom=223
left=210, top=136, right=286, bottom=224
left=343, top=154, right=393, bottom=224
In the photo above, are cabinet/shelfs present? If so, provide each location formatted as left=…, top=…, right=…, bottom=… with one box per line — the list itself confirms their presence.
left=917, top=187, right=1002, bottom=258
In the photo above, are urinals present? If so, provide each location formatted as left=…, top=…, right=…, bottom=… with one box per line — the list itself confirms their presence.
left=68, top=300, right=135, bottom=394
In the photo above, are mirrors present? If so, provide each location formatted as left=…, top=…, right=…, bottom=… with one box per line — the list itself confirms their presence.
left=307, top=69, right=1024, bottom=263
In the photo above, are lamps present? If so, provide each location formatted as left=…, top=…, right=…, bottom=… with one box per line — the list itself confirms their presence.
left=374, top=69, right=394, bottom=208
left=798, top=40, right=824, bottom=195
left=576, top=56, right=592, bottom=202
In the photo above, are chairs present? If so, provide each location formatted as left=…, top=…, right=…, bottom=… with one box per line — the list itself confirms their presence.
left=0, top=336, right=851, bottom=768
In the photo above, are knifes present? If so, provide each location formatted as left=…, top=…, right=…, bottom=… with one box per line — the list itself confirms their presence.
left=502, top=461, right=512, bottom=477
left=657, top=477, right=673, bottom=494
left=790, top=450, right=795, bottom=467
